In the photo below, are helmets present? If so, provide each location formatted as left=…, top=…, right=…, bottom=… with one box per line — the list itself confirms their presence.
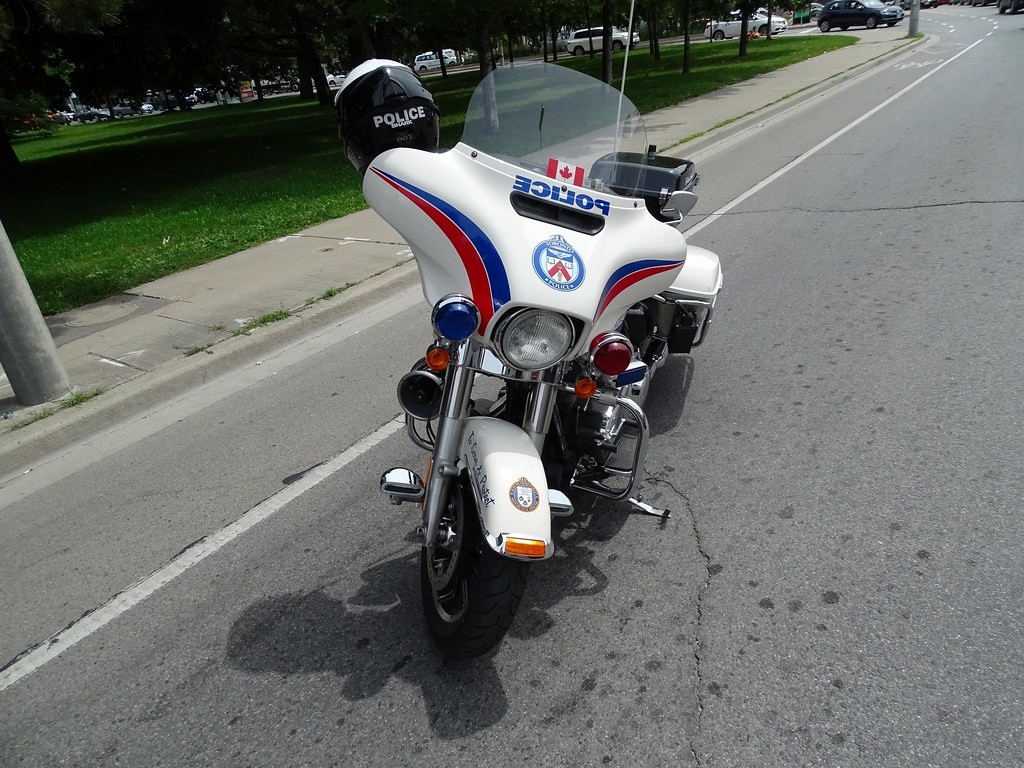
left=333, top=57, right=441, bottom=172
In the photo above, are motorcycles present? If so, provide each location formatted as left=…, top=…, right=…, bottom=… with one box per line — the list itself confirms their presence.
left=361, top=55, right=729, bottom=658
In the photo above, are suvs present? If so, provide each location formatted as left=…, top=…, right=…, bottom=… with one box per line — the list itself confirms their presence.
left=564, top=26, right=641, bottom=57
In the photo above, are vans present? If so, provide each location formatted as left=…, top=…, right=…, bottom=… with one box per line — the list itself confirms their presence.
left=414, top=49, right=467, bottom=74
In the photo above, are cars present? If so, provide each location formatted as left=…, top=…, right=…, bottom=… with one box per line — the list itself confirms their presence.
left=703, top=9, right=789, bottom=40
left=817, top=0, right=906, bottom=33
left=883, top=0, right=1024, bottom=16
left=251, top=71, right=348, bottom=96
left=10, top=85, right=217, bottom=128
